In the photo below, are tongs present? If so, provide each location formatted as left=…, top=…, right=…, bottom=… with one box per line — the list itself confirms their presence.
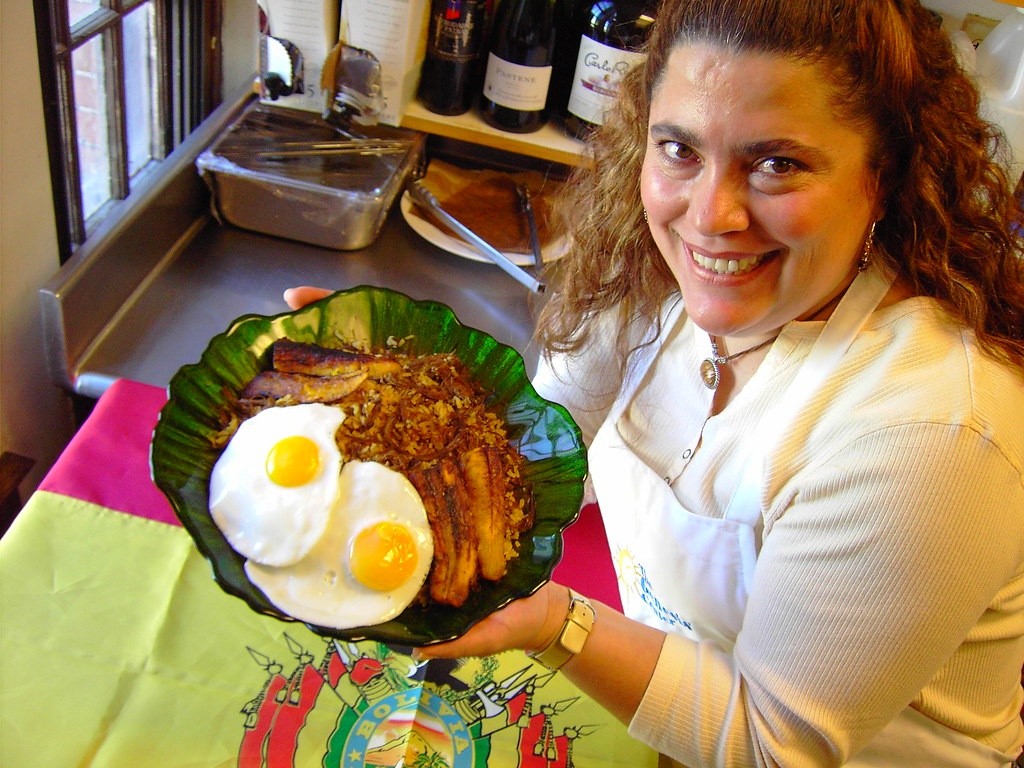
left=407, top=176, right=548, bottom=296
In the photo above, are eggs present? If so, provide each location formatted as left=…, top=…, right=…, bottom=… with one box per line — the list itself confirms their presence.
left=209, top=403, right=346, bottom=567
left=243, top=459, right=434, bottom=630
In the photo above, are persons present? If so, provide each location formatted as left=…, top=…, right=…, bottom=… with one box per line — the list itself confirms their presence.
left=283, top=1, right=1023, bottom=768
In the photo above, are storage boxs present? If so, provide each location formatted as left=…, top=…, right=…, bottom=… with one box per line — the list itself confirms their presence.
left=197, top=104, right=424, bottom=251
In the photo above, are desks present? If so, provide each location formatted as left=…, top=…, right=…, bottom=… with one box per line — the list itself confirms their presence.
left=0, top=380, right=659, bottom=768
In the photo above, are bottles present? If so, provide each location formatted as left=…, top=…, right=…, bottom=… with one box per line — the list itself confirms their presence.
left=560, top=0, right=661, bottom=142
left=419, top=0, right=495, bottom=116
left=479, top=0, right=556, bottom=133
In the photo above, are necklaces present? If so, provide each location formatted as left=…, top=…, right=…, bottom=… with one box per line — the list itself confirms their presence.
left=699, top=277, right=856, bottom=390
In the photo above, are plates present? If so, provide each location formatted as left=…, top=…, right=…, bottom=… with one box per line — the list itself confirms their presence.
left=149, top=285, right=588, bottom=646
left=399, top=169, right=575, bottom=266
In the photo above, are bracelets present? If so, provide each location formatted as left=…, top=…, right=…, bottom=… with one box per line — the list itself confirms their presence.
left=526, top=588, right=596, bottom=673
